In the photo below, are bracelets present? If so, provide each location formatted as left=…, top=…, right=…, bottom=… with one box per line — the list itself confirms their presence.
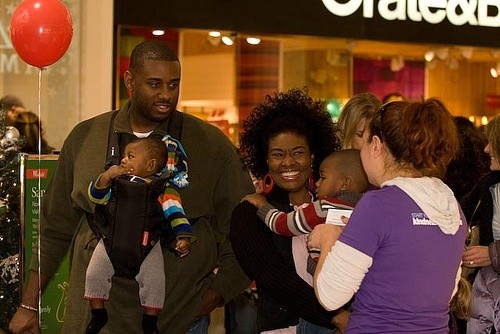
left=21, top=303, right=38, bottom=312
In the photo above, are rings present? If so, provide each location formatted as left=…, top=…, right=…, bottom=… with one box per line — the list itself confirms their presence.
left=469, top=261, right=473, bottom=264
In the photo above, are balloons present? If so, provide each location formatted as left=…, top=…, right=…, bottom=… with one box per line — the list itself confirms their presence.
left=9, top=0, right=74, bottom=70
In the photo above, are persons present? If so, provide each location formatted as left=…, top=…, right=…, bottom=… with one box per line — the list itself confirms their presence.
left=83, top=136, right=194, bottom=334
left=230, top=86, right=354, bottom=333
left=305, top=96, right=467, bottom=334
left=240, top=149, right=372, bottom=277
left=334, top=92, right=384, bottom=149
left=11, top=111, right=56, bottom=155
left=461, top=115, right=500, bottom=334
left=8, top=41, right=256, bottom=334
